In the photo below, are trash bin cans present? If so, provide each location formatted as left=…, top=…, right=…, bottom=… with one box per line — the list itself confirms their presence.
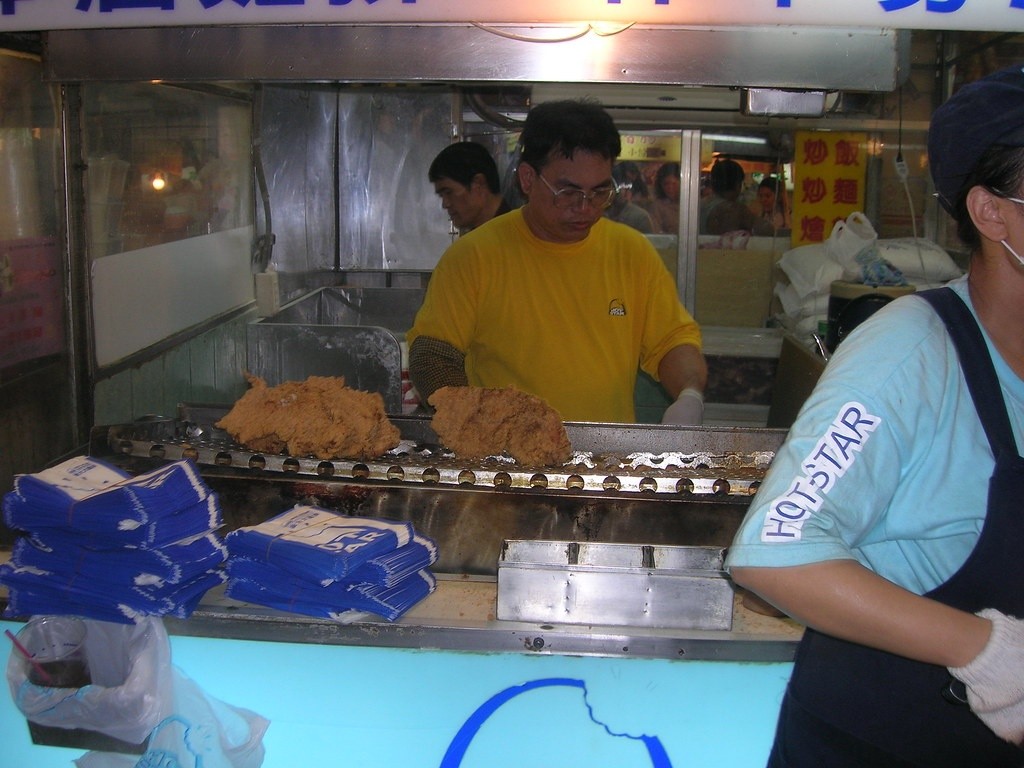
left=5, top=613, right=175, bottom=755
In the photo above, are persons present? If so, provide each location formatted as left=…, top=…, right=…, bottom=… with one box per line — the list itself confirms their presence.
left=596, top=155, right=784, bottom=235
left=722, top=65, right=1024, bottom=768
left=426, top=142, right=512, bottom=235
left=406, top=98, right=708, bottom=424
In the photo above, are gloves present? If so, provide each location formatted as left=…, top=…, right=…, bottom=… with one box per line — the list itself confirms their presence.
left=947, top=608, right=1024, bottom=746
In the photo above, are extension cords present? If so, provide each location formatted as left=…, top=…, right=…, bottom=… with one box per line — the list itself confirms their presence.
left=893, top=154, right=909, bottom=179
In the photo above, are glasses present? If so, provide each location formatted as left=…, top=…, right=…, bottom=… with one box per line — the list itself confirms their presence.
left=540, top=175, right=620, bottom=210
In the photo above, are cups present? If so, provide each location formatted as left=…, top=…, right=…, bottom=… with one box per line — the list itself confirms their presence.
left=13, top=614, right=92, bottom=687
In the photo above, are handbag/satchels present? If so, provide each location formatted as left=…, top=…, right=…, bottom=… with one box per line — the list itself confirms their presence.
left=823, top=212, right=908, bottom=287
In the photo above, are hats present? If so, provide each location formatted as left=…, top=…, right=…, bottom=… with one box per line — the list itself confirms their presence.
left=927, top=64, right=1024, bottom=211
